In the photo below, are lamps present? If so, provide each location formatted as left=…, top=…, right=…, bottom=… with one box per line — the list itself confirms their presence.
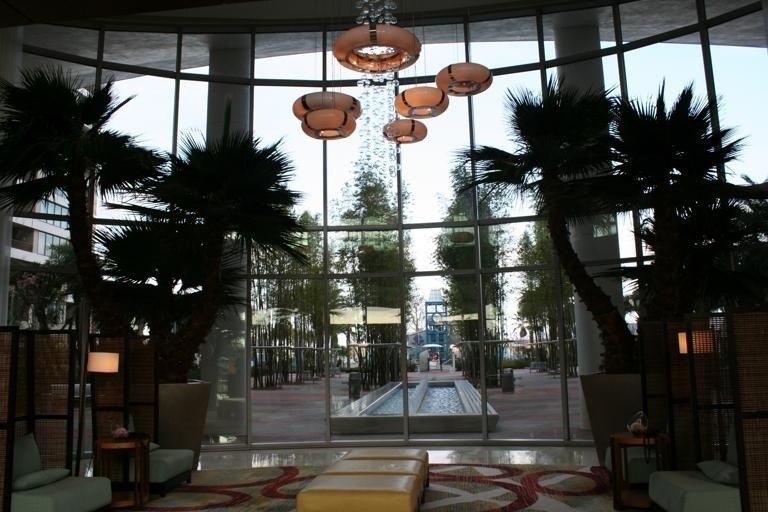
left=88, top=351, right=119, bottom=372
left=292, top=0, right=495, bottom=144
left=677, top=329, right=714, bottom=354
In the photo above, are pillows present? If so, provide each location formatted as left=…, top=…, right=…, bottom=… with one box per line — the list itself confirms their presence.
left=150, top=441, right=161, bottom=453
left=696, top=459, right=739, bottom=486
left=12, top=468, right=71, bottom=490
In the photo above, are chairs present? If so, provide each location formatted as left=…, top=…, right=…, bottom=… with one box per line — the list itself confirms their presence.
left=604, top=422, right=668, bottom=490
left=648, top=412, right=742, bottom=512
left=12, top=433, right=113, bottom=512
left=128, top=439, right=195, bottom=498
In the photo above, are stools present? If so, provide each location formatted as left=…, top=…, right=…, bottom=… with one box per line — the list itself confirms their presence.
left=294, top=445, right=431, bottom=512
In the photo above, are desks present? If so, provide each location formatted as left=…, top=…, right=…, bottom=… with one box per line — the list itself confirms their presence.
left=97, top=438, right=150, bottom=507
left=609, top=433, right=662, bottom=511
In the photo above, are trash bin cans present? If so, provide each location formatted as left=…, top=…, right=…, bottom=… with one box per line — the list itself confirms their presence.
left=348, top=373, right=362, bottom=401
left=502, top=368, right=514, bottom=394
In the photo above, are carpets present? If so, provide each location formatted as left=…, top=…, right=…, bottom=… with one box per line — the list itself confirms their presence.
left=94, top=463, right=612, bottom=512
